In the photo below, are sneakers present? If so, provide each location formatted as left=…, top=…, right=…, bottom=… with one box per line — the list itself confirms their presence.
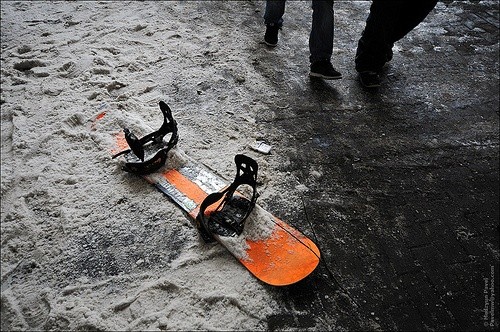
left=310, top=62, right=342, bottom=79
left=264, top=27, right=278, bottom=47
left=361, top=73, right=381, bottom=87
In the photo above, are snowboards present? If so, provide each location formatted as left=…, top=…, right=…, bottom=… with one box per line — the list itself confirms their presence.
left=88, top=109, right=322, bottom=286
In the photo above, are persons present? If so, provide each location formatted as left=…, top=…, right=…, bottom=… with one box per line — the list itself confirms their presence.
left=355, top=0, right=438, bottom=88
left=263, top=0, right=342, bottom=80
